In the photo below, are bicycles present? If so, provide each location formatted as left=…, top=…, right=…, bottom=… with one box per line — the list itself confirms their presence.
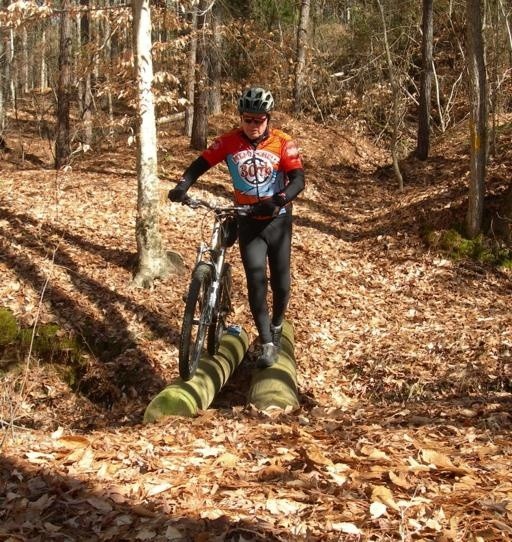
left=168, top=193, right=269, bottom=382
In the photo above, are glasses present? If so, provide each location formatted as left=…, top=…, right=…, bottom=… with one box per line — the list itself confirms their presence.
left=241, top=113, right=267, bottom=125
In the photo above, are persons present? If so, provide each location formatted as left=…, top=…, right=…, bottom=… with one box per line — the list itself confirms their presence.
left=168, top=86, right=304, bottom=369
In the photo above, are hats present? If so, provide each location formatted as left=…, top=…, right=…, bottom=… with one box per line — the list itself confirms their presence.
left=238, top=87, right=276, bottom=115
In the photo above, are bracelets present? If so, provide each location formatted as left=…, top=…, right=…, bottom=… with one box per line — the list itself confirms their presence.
left=179, top=177, right=189, bottom=190
left=278, top=191, right=288, bottom=204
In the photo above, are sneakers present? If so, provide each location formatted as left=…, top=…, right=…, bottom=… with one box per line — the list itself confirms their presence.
left=258, top=344, right=279, bottom=368
left=269, top=325, right=283, bottom=345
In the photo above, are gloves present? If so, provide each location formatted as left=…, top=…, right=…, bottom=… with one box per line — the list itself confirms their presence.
left=252, top=195, right=288, bottom=221
left=168, top=187, right=190, bottom=204
